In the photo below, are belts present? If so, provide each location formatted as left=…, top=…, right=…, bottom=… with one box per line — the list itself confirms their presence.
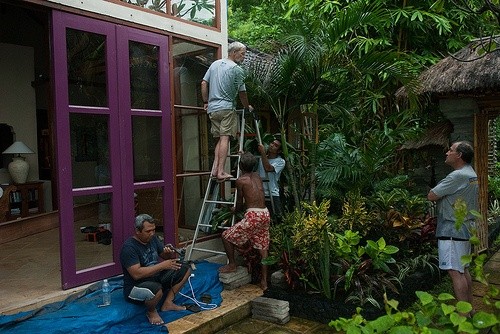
left=438, top=236, right=470, bottom=241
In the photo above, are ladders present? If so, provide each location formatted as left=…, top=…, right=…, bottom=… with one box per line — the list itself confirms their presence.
left=187, top=107, right=278, bottom=263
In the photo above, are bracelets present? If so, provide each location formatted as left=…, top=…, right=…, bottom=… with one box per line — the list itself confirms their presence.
left=203, top=102, right=208, bottom=104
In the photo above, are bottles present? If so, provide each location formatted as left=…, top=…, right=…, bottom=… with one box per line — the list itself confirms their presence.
left=102, top=279, right=111, bottom=306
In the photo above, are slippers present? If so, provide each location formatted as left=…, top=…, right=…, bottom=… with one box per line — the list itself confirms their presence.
left=212, top=175, right=234, bottom=183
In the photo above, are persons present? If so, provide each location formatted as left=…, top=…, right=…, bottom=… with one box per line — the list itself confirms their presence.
left=201, top=41, right=255, bottom=183
left=428, top=141, right=479, bottom=319
left=256, top=138, right=285, bottom=223
left=119, top=213, right=192, bottom=326
left=218, top=152, right=271, bottom=291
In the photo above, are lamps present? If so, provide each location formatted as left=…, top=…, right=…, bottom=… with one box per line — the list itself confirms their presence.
left=2, top=141, right=36, bottom=183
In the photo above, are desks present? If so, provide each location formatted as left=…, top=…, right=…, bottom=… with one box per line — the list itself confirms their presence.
left=0, top=182, right=45, bottom=217
